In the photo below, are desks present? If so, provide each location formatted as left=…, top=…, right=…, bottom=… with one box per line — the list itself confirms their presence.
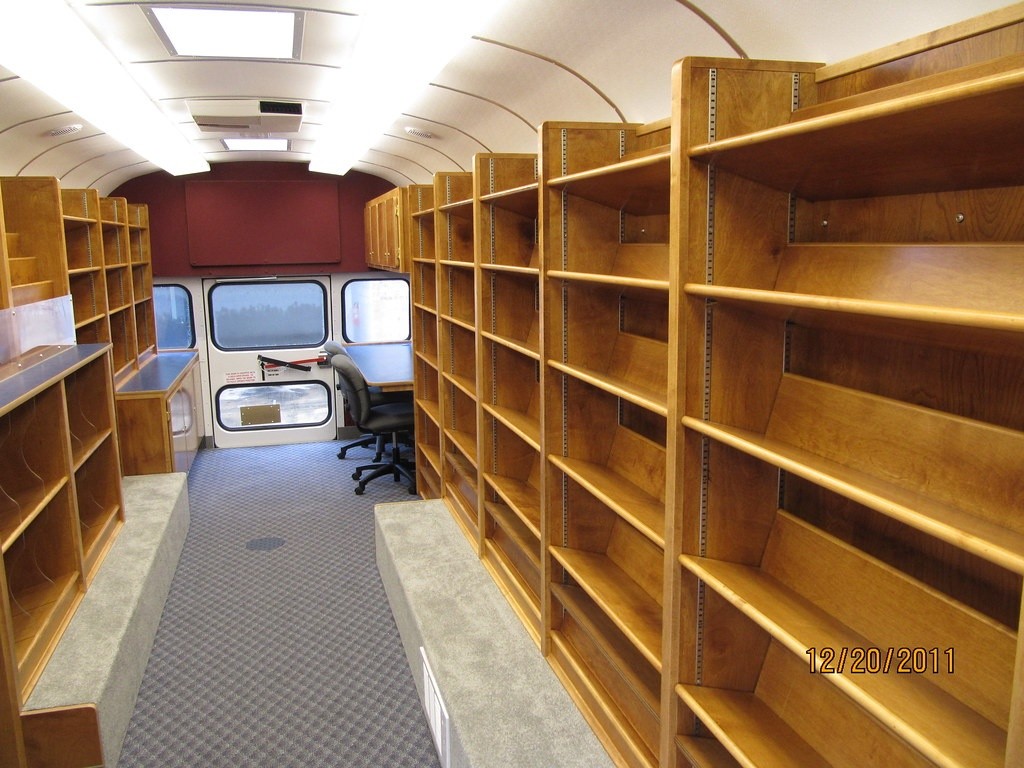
left=343, top=342, right=413, bottom=391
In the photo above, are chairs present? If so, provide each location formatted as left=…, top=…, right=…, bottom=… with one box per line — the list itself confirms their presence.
left=324, top=340, right=417, bottom=496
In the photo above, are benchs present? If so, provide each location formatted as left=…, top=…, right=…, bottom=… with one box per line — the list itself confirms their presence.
left=372, top=498, right=616, bottom=768
left=18, top=471, right=190, bottom=766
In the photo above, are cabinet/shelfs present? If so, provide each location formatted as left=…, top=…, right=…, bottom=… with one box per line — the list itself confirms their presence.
left=1, top=175, right=205, bottom=768
left=364, top=2, right=1024, bottom=766
